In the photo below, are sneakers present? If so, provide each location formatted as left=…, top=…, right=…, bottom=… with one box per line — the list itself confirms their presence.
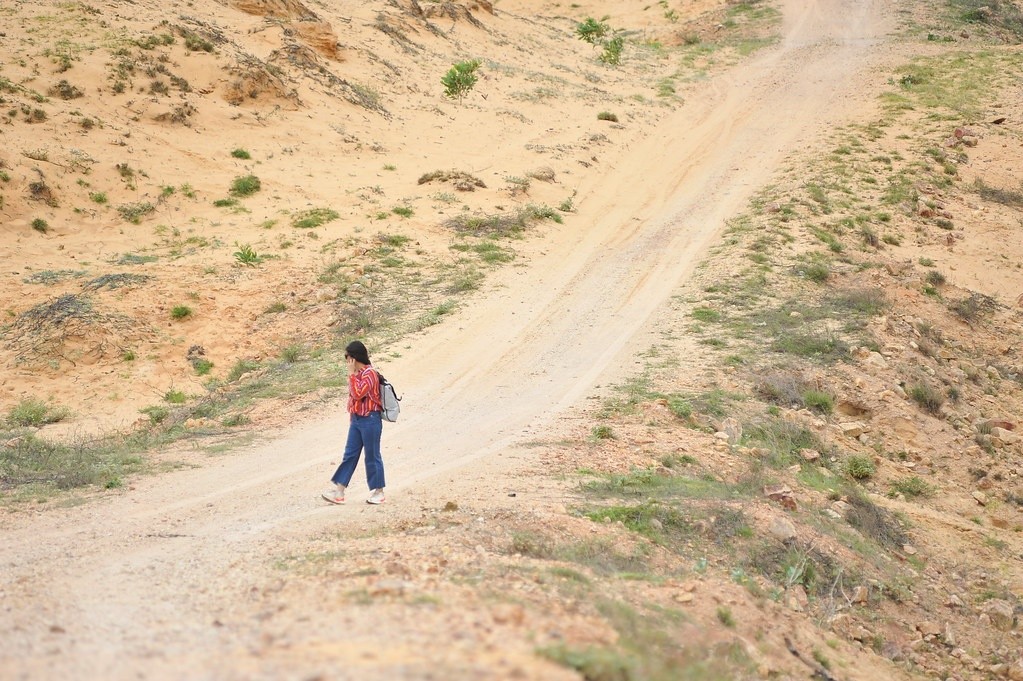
left=366, top=492, right=385, bottom=504
left=321, top=489, right=345, bottom=504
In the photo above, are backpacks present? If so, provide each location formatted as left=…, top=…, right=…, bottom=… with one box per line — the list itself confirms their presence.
left=362, top=367, right=401, bottom=422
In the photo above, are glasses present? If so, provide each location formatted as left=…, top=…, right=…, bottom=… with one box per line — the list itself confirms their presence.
left=345, top=354, right=350, bottom=359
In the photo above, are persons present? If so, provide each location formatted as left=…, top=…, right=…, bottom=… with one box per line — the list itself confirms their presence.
left=321, top=341, right=386, bottom=504
